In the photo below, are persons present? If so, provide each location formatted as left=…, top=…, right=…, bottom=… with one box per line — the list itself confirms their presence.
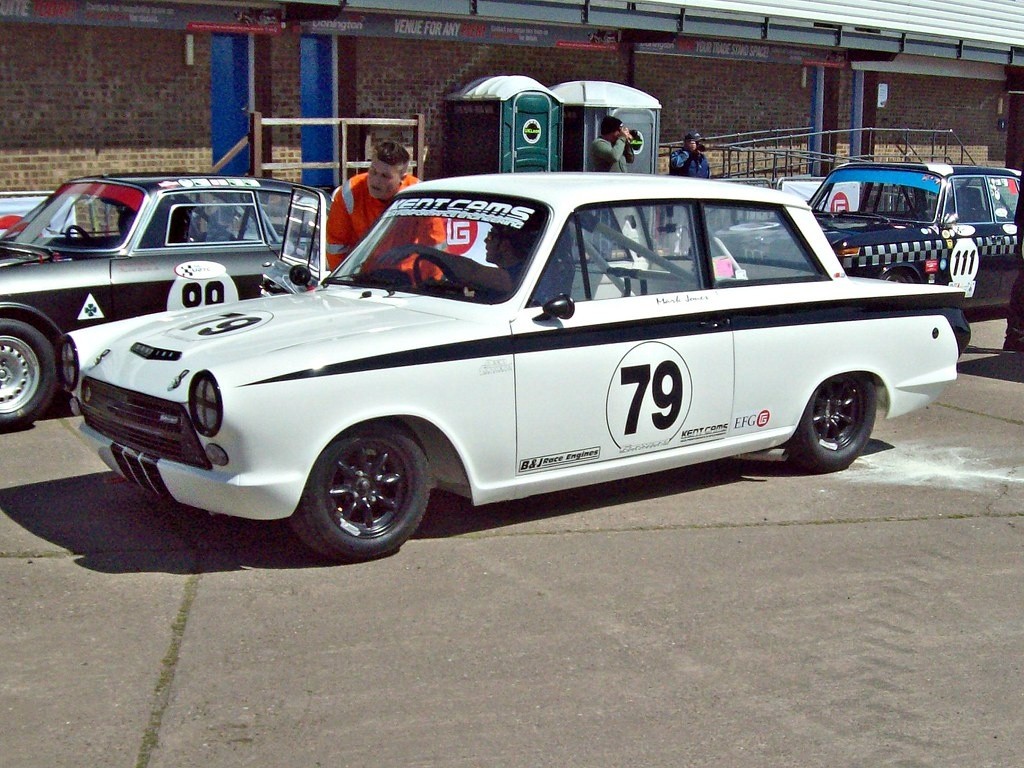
left=377, top=221, right=549, bottom=305
left=586, top=117, right=635, bottom=261
left=668, top=132, right=711, bottom=256
left=324, top=141, right=445, bottom=285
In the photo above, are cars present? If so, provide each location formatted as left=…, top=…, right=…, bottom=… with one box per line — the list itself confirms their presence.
left=0, top=169, right=338, bottom=434
left=729, top=161, right=1024, bottom=315
left=55, top=170, right=972, bottom=566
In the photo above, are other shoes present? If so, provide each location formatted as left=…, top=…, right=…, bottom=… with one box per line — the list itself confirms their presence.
left=1003, top=335, right=1024, bottom=351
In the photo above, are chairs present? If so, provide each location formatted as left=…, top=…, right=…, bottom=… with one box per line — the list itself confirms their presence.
left=547, top=248, right=576, bottom=297
left=949, top=187, right=983, bottom=223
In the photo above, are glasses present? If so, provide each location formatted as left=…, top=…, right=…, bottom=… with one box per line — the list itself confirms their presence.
left=487, top=232, right=508, bottom=241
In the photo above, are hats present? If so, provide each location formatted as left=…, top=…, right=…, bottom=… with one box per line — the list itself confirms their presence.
left=601, top=115, right=623, bottom=135
left=684, top=132, right=706, bottom=142
